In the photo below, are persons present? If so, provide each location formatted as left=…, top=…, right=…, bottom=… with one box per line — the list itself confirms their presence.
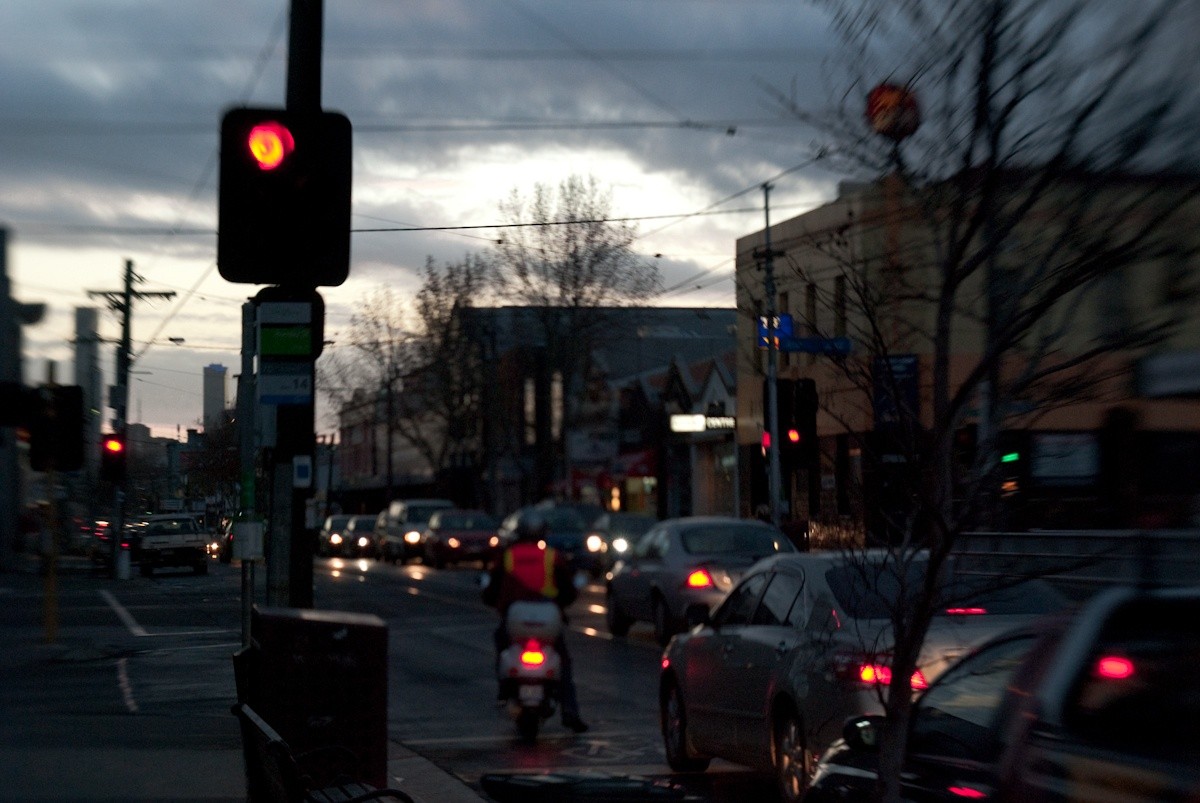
left=487, top=509, right=592, bottom=734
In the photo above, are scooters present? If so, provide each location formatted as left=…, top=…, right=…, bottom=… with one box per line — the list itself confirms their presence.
left=475, top=569, right=592, bottom=750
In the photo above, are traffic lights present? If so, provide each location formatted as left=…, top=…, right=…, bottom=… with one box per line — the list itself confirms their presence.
left=216, top=106, right=356, bottom=289
left=787, top=377, right=819, bottom=454
left=992, top=429, right=1034, bottom=514
left=97, top=434, right=127, bottom=484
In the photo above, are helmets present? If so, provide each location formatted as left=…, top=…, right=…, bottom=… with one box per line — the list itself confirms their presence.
left=520, top=513, right=548, bottom=535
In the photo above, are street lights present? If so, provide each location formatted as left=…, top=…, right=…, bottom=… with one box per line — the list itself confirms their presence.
left=112, top=335, right=187, bottom=532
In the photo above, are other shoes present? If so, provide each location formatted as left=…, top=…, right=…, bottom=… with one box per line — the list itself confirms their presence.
left=562, top=716, right=590, bottom=733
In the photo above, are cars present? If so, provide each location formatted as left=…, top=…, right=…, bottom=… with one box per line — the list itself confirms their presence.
left=802, top=615, right=1058, bottom=803
left=987, top=576, right=1200, bottom=803
left=317, top=497, right=658, bottom=583
left=604, top=513, right=805, bottom=650
left=650, top=544, right=1054, bottom=803
left=102, top=512, right=272, bottom=575
